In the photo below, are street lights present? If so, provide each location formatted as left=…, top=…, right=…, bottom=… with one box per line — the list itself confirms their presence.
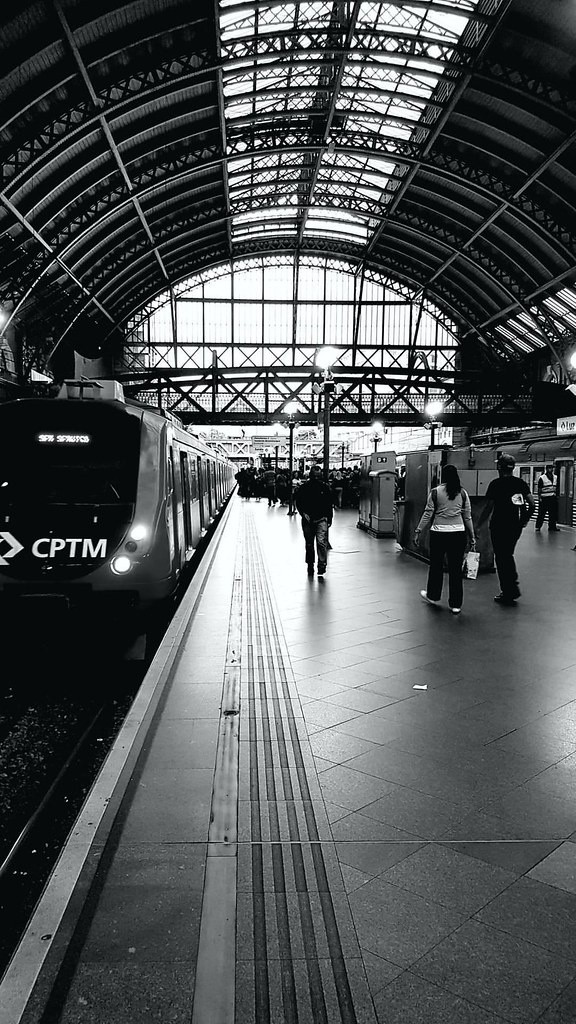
left=315, top=346, right=341, bottom=484
left=283, top=402, right=298, bottom=516
left=370, top=422, right=383, bottom=453
left=272, top=423, right=284, bottom=472
left=424, top=401, right=445, bottom=452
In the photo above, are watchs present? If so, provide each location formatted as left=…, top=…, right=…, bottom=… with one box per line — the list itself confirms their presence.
left=414, top=529, right=421, bottom=533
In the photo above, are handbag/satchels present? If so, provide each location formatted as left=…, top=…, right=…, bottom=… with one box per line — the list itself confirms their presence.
left=461, top=544, right=480, bottom=580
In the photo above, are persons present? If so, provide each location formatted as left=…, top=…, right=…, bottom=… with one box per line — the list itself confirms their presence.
left=295, top=465, right=334, bottom=575
left=477, top=453, right=536, bottom=602
left=233, top=461, right=361, bottom=513
left=535, top=464, right=559, bottom=532
left=413, top=463, right=478, bottom=613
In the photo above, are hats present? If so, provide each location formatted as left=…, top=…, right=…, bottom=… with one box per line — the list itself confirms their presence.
left=546, top=465, right=555, bottom=468
left=494, top=452, right=517, bottom=463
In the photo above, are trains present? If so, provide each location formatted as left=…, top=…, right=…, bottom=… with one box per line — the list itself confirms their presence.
left=430, top=415, right=575, bottom=528
left=0, top=372, right=239, bottom=626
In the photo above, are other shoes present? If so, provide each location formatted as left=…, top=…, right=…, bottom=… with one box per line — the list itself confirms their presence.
left=446, top=602, right=463, bottom=613
left=317, top=565, right=327, bottom=574
left=307, top=567, right=315, bottom=575
left=548, top=525, right=560, bottom=531
left=493, top=590, right=521, bottom=602
left=534, top=525, right=541, bottom=531
left=419, top=589, right=442, bottom=605
